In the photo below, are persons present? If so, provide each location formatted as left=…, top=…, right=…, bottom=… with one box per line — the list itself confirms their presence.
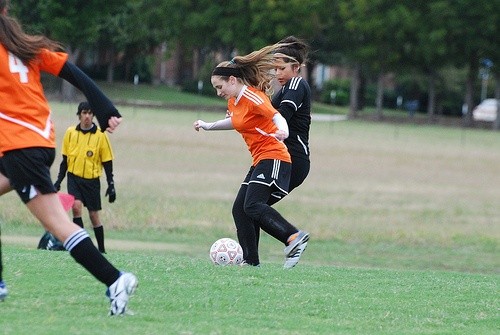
left=54, top=101, right=116, bottom=254
left=194, top=42, right=310, bottom=270
left=0, top=0, right=137, bottom=316
left=253, top=36, right=310, bottom=250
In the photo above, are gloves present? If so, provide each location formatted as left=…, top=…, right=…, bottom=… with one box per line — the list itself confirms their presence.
left=105, top=180, right=117, bottom=203
left=54, top=181, right=60, bottom=191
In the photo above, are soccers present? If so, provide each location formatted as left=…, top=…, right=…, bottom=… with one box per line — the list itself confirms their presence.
left=210, top=238, right=244, bottom=266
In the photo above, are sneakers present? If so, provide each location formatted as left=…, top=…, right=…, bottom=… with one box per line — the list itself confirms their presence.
left=105, top=272, right=139, bottom=316
left=238, top=260, right=261, bottom=267
left=284, top=230, right=310, bottom=269
left=0, top=282, right=7, bottom=300
